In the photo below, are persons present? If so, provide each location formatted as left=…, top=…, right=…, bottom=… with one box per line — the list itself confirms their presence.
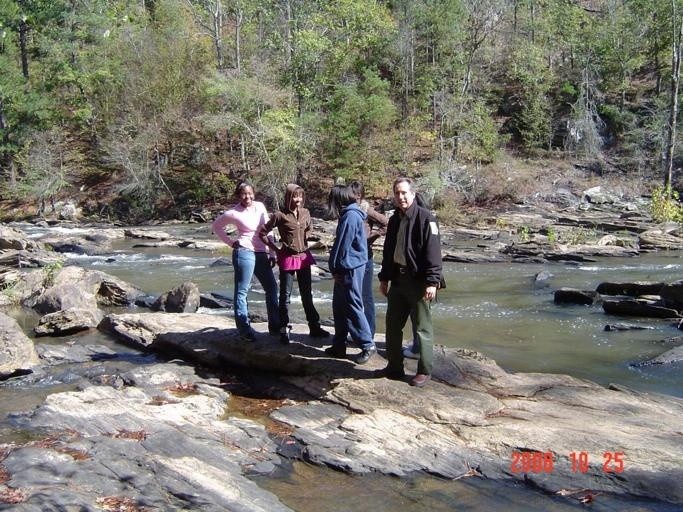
left=373, top=176, right=447, bottom=387
left=324, top=185, right=377, bottom=364
left=259, top=184, right=329, bottom=344
left=212, top=182, right=292, bottom=341
left=402, top=191, right=425, bottom=360
left=347, top=180, right=388, bottom=340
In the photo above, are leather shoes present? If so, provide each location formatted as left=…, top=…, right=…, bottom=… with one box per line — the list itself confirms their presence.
left=375, top=365, right=405, bottom=379
left=280, top=326, right=291, bottom=343
left=237, top=322, right=255, bottom=342
left=325, top=343, right=347, bottom=357
left=353, top=345, right=377, bottom=364
left=309, top=322, right=330, bottom=337
left=410, top=373, right=431, bottom=387
